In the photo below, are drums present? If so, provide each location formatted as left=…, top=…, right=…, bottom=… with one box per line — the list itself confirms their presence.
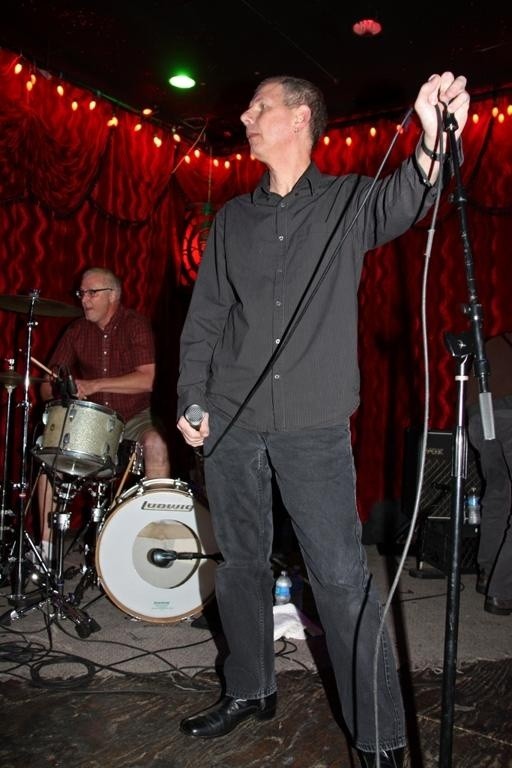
left=95, top=480, right=220, bottom=625
left=32, top=397, right=124, bottom=481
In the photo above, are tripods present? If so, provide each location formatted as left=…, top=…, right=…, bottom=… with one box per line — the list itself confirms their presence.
left=0, top=310, right=101, bottom=638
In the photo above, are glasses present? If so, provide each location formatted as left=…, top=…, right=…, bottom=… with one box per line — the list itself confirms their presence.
left=76, top=287, right=114, bottom=297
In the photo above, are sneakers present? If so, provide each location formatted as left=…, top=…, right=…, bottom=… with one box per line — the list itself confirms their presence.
left=483, top=596, right=512, bottom=615
left=475, top=568, right=490, bottom=596
left=26, top=544, right=53, bottom=573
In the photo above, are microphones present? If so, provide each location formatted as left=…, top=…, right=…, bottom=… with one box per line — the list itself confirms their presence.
left=185, top=404, right=203, bottom=452
left=151, top=548, right=173, bottom=568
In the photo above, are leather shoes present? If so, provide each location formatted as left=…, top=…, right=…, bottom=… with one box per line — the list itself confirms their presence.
left=180, top=689, right=277, bottom=740
left=362, top=747, right=407, bottom=768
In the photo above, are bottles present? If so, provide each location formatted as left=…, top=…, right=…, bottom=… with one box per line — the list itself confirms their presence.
left=466, top=486, right=482, bottom=525
left=273, top=569, right=293, bottom=606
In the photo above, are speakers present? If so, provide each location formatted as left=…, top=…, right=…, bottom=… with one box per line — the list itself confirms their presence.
left=403, top=429, right=487, bottom=521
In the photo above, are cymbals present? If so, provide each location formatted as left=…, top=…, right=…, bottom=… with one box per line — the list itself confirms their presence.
left=0, top=371, right=52, bottom=386
left=0, top=295, right=83, bottom=319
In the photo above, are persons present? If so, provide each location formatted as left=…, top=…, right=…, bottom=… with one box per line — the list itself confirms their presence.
left=462, top=317, right=512, bottom=615
left=166, top=65, right=478, bottom=766
left=22, top=261, right=174, bottom=571
